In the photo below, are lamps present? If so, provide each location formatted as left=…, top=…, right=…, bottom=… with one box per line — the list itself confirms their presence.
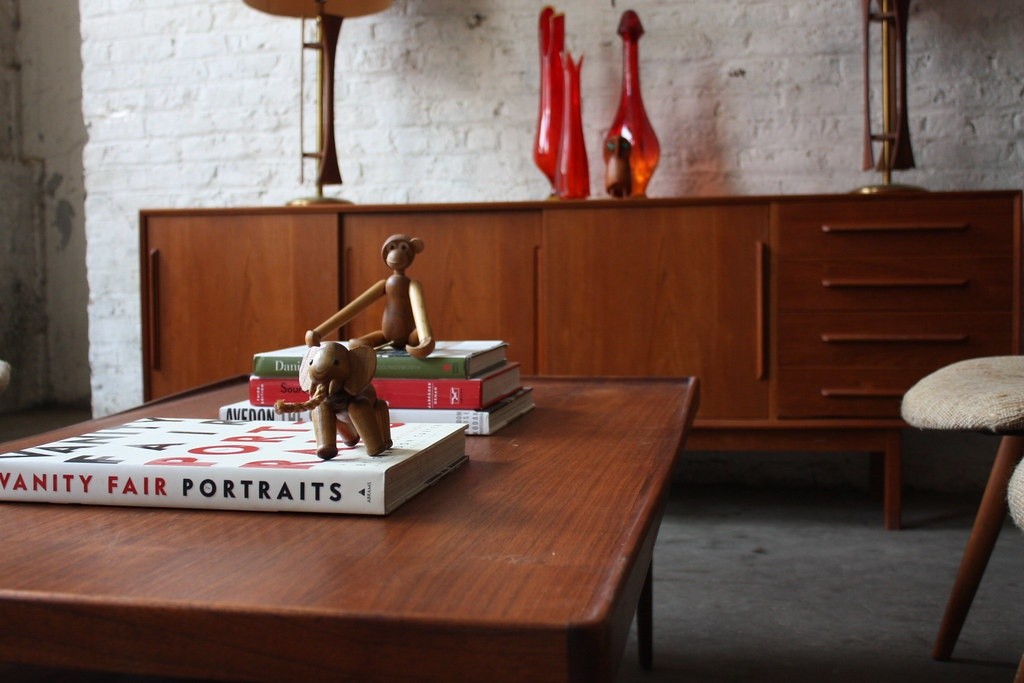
left=241, top=0, right=395, bottom=208
left=850, top=0, right=932, bottom=196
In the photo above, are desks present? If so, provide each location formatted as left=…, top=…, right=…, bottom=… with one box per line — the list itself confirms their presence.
left=0, top=371, right=704, bottom=683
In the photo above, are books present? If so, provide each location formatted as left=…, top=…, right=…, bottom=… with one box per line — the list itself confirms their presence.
left=250, top=362, right=523, bottom=409
left=253, top=340, right=509, bottom=379
left=0, top=418, right=472, bottom=515
left=219, top=387, right=536, bottom=435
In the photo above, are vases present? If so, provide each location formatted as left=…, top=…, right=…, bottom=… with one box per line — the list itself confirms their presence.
left=530, top=5, right=592, bottom=201
left=602, top=8, right=665, bottom=201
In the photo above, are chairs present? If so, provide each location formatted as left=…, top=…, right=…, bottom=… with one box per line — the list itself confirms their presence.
left=901, top=353, right=1024, bottom=683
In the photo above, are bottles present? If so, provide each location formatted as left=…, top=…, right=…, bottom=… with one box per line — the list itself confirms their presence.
left=556, top=51, right=591, bottom=199
left=602, top=9, right=660, bottom=196
left=533, top=5, right=565, bottom=200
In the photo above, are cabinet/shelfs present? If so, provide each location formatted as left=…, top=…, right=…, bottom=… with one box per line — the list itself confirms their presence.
left=133, top=189, right=1024, bottom=683
